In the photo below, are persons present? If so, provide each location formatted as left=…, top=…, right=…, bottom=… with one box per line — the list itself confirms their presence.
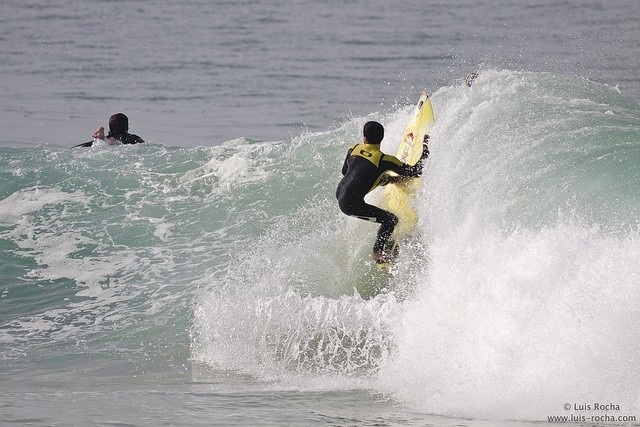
left=336, top=120, right=429, bottom=264
left=69, top=112, right=146, bottom=149
left=463, top=69, right=484, bottom=88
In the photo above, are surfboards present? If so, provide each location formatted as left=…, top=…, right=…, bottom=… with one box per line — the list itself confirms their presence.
left=375, top=90, right=436, bottom=270
